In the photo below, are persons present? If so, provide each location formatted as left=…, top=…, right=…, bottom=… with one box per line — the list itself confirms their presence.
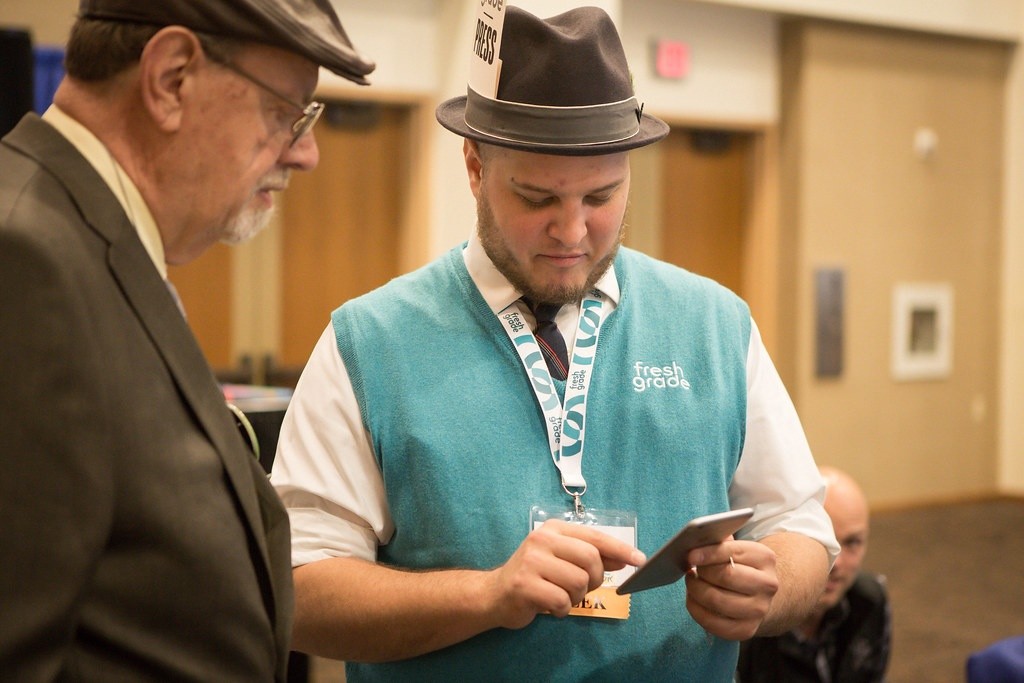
left=269, top=2, right=841, bottom=683
left=1, top=0, right=376, bottom=682
left=732, top=468, right=894, bottom=682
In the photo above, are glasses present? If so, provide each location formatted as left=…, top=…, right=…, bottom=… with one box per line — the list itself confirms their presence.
left=199, top=41, right=326, bottom=150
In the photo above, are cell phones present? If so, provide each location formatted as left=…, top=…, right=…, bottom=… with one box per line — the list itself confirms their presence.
left=616, top=507, right=755, bottom=596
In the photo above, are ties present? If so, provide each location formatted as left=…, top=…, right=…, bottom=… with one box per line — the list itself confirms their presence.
left=519, top=292, right=569, bottom=381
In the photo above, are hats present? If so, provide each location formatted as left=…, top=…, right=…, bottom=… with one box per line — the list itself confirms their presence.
left=80, top=1, right=375, bottom=87
left=435, top=0, right=670, bottom=155
